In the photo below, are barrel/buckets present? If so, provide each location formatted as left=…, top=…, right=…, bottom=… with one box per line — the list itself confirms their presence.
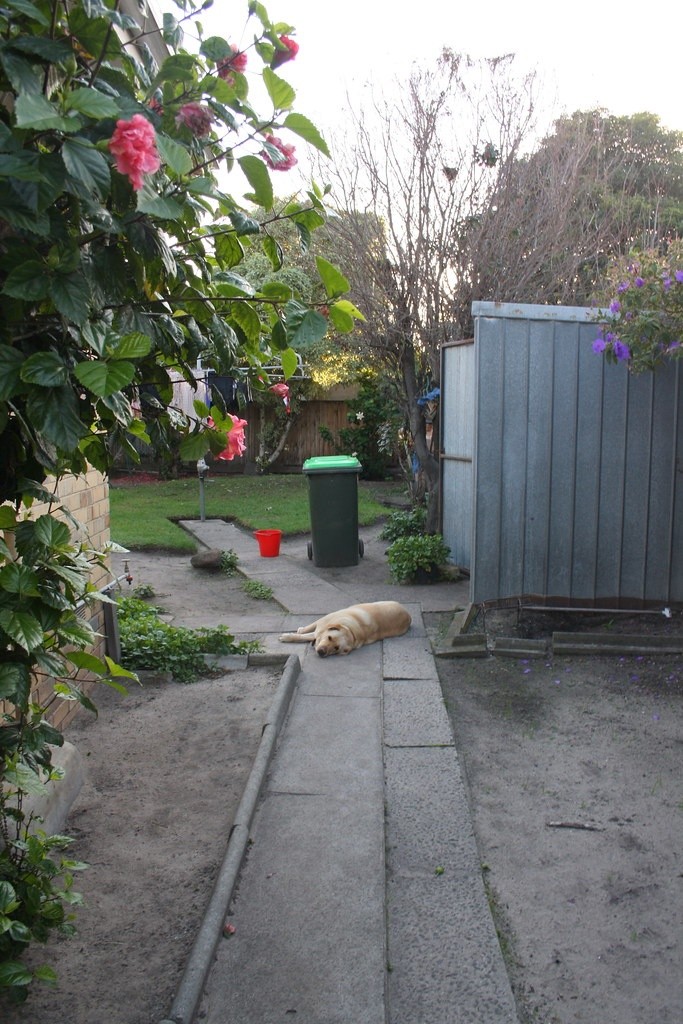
left=253, top=529, right=283, bottom=559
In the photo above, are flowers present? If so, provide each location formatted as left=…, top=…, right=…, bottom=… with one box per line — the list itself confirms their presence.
left=590, top=262, right=682, bottom=363
left=90, top=29, right=302, bottom=460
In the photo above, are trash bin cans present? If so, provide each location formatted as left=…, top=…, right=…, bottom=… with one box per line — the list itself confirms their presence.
left=303, top=455, right=366, bottom=568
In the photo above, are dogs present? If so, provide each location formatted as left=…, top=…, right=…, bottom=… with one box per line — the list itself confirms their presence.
left=278, top=600, right=412, bottom=659
